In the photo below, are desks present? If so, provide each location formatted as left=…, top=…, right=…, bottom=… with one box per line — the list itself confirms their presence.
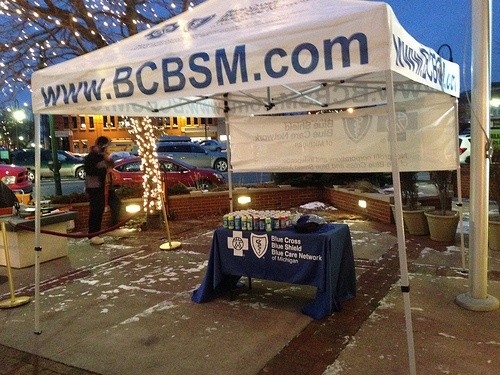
left=191, top=225, right=358, bottom=321
left=0, top=208, right=77, bottom=269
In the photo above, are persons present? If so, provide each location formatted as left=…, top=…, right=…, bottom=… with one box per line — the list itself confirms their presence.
left=83, top=136, right=122, bottom=244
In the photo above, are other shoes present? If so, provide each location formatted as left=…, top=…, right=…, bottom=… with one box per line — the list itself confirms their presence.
left=88, top=236, right=105, bottom=244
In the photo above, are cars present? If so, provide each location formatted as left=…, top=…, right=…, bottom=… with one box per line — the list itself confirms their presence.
left=112, top=156, right=227, bottom=196
left=157, top=140, right=228, bottom=172
left=11, top=150, right=87, bottom=182
left=0, top=163, right=35, bottom=200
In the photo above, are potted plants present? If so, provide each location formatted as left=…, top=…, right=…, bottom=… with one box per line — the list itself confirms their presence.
left=389, top=172, right=422, bottom=232
left=424, top=170, right=460, bottom=243
left=487, top=139, right=500, bottom=252
left=401, top=171, right=429, bottom=236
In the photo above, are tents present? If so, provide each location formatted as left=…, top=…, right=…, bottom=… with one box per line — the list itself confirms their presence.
left=34, top=0, right=465, bottom=375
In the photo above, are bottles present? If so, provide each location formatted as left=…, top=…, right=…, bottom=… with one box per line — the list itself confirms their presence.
left=223, top=209, right=297, bottom=232
